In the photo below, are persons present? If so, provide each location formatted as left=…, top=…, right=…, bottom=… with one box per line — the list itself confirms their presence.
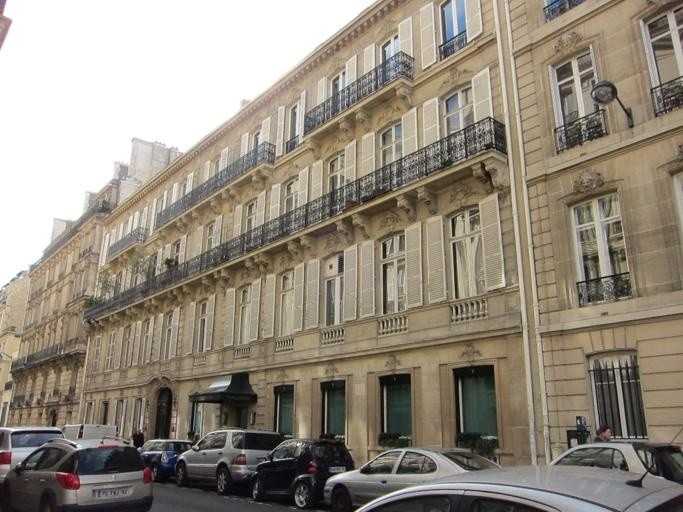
left=132, top=427, right=145, bottom=450
left=591, top=425, right=613, bottom=444
left=186, top=427, right=195, bottom=440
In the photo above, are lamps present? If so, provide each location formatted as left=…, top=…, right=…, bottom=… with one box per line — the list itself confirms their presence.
left=590, top=79, right=635, bottom=132
